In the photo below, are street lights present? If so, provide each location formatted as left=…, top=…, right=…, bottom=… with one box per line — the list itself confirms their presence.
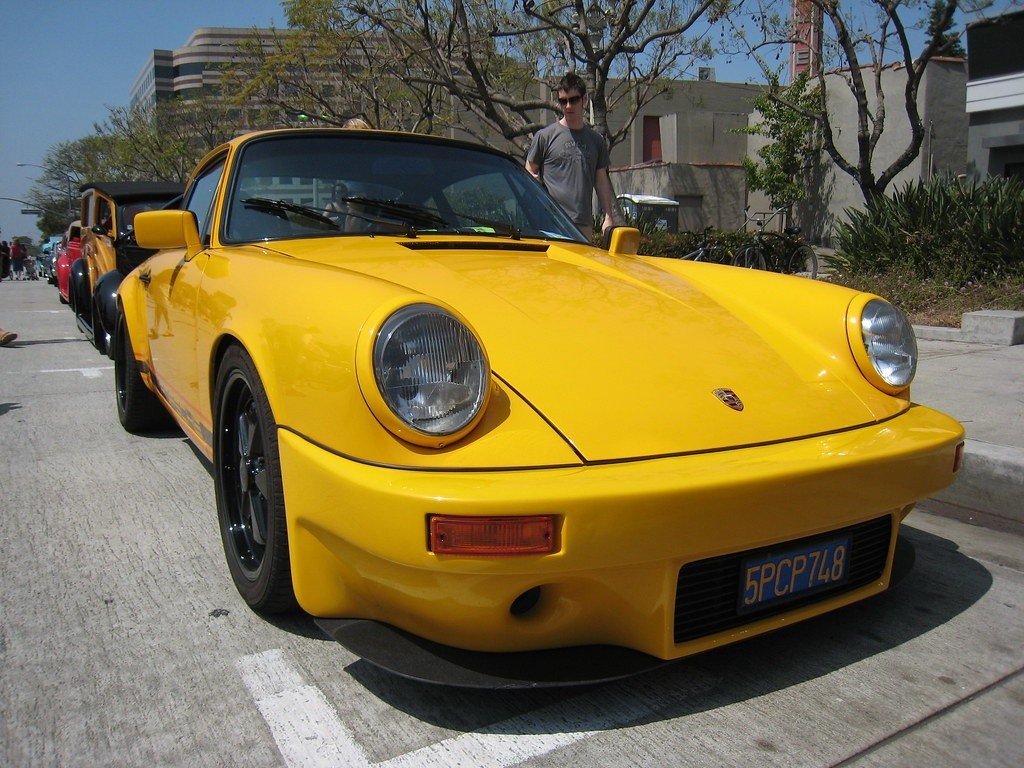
left=572, top=7, right=622, bottom=224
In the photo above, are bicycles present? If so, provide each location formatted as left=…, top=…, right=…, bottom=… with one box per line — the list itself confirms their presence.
left=678, top=225, right=728, bottom=264
left=730, top=204, right=818, bottom=279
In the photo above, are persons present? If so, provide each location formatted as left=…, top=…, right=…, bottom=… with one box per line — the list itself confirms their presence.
left=0, top=327, right=18, bottom=346
left=525, top=72, right=614, bottom=243
left=0, top=238, right=27, bottom=281
left=320, top=183, right=349, bottom=230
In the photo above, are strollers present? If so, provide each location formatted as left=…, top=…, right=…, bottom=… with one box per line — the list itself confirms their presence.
left=24, top=260, right=39, bottom=280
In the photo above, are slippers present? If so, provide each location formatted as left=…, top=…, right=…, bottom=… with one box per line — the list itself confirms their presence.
left=0, top=331, right=18, bottom=345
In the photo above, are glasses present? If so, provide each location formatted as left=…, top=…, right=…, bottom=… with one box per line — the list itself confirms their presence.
left=335, top=190, right=347, bottom=195
left=557, top=94, right=584, bottom=106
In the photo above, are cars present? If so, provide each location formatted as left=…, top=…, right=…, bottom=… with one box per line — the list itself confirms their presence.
left=68, top=181, right=187, bottom=360
left=36, top=219, right=81, bottom=304
left=95, top=117, right=967, bottom=691
